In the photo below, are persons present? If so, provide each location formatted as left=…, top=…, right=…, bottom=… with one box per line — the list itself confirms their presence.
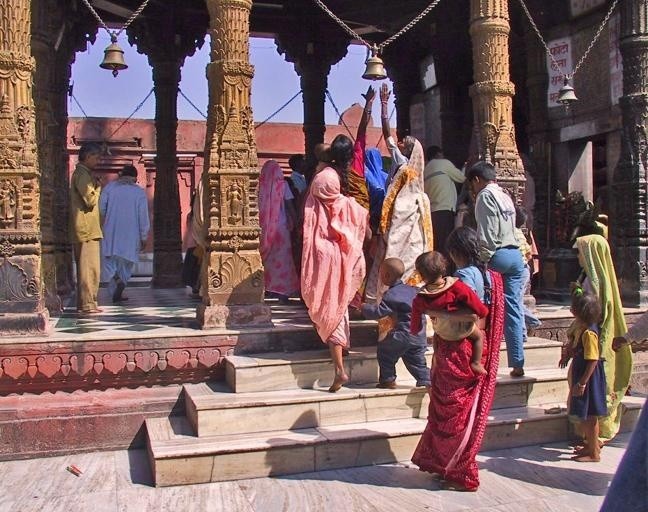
left=98, top=165, right=150, bottom=303
left=68, top=142, right=104, bottom=314
left=558, top=234, right=633, bottom=462
left=598, top=312, right=648, bottom=512
left=508, top=189, right=541, bottom=336
left=259, top=82, right=525, bottom=392
left=410, top=225, right=504, bottom=490
